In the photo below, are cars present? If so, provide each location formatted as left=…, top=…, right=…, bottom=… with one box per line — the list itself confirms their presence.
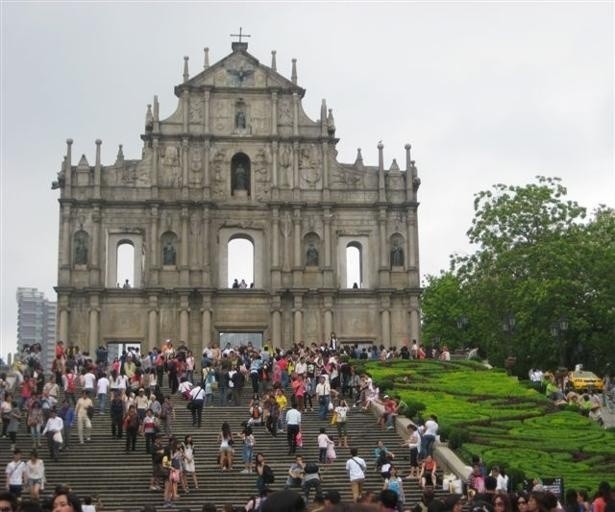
left=564, top=367, right=605, bottom=393
left=552, top=366, right=569, bottom=379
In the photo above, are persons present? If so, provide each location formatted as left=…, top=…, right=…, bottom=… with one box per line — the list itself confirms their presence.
left=162, top=240, right=177, bottom=265
left=0, top=332, right=615, bottom=512
left=75, top=240, right=88, bottom=263
left=390, top=239, right=404, bottom=265
left=305, top=243, right=319, bottom=264
left=123, top=279, right=130, bottom=289
left=232, top=278, right=254, bottom=289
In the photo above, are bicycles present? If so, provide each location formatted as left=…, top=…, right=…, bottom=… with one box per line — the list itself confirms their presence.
left=596, top=383, right=615, bottom=414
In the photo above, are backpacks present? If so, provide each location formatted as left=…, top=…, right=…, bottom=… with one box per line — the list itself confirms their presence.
left=66, top=374, right=74, bottom=393
left=263, top=466, right=274, bottom=483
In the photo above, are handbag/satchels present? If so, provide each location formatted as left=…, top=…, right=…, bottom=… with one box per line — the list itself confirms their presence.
left=187, top=402, right=193, bottom=409
